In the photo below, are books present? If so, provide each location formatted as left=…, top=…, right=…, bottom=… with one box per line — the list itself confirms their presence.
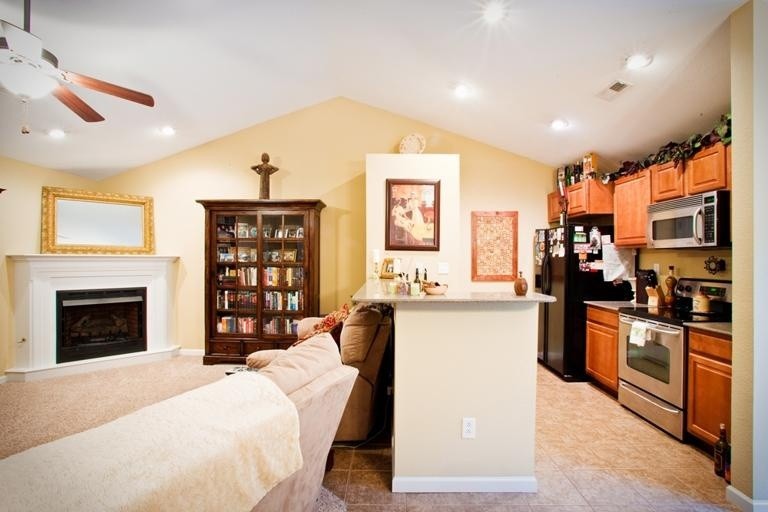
left=216, top=223, right=305, bottom=335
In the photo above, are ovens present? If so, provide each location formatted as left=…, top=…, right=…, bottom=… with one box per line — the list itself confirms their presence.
left=619, top=312, right=687, bottom=442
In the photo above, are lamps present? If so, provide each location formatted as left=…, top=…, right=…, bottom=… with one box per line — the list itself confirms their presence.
left=0, top=54, right=59, bottom=104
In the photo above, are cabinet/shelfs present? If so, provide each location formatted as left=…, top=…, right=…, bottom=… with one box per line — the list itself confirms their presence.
left=547, top=180, right=613, bottom=223
left=196, top=199, right=327, bottom=365
left=613, top=140, right=732, bottom=248
left=688, top=326, right=732, bottom=449
left=585, top=304, right=618, bottom=401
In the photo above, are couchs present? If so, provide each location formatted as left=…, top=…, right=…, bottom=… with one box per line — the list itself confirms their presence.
left=246, top=316, right=392, bottom=449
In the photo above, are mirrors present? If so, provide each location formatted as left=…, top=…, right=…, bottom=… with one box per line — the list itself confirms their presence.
left=41, top=184, right=155, bottom=255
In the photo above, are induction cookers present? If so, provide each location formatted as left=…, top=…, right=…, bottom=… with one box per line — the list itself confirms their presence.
left=621, top=308, right=718, bottom=325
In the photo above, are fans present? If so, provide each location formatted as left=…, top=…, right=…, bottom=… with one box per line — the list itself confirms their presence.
left=0, top=0, right=154, bottom=122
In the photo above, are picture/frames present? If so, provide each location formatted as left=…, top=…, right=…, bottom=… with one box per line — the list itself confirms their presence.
left=384, top=178, right=440, bottom=250
left=471, top=210, right=518, bottom=281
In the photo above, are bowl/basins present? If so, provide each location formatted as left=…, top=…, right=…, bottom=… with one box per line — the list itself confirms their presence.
left=425, top=286, right=446, bottom=295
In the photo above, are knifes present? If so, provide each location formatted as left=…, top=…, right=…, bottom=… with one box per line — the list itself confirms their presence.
left=644, top=270, right=659, bottom=288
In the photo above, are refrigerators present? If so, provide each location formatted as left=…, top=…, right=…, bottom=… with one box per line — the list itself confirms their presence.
left=535, top=224, right=631, bottom=381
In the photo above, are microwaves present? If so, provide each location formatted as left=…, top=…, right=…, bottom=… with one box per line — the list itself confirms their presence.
left=646, top=190, right=730, bottom=251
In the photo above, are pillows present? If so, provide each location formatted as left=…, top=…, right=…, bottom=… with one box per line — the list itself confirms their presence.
left=258, top=332, right=343, bottom=395
left=340, top=303, right=383, bottom=365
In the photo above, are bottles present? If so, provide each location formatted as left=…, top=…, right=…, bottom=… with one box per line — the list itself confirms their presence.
left=557, top=160, right=584, bottom=186
left=665, top=265, right=676, bottom=307
left=714, top=422, right=728, bottom=475
left=513, top=270, right=527, bottom=296
left=414, top=268, right=420, bottom=283
